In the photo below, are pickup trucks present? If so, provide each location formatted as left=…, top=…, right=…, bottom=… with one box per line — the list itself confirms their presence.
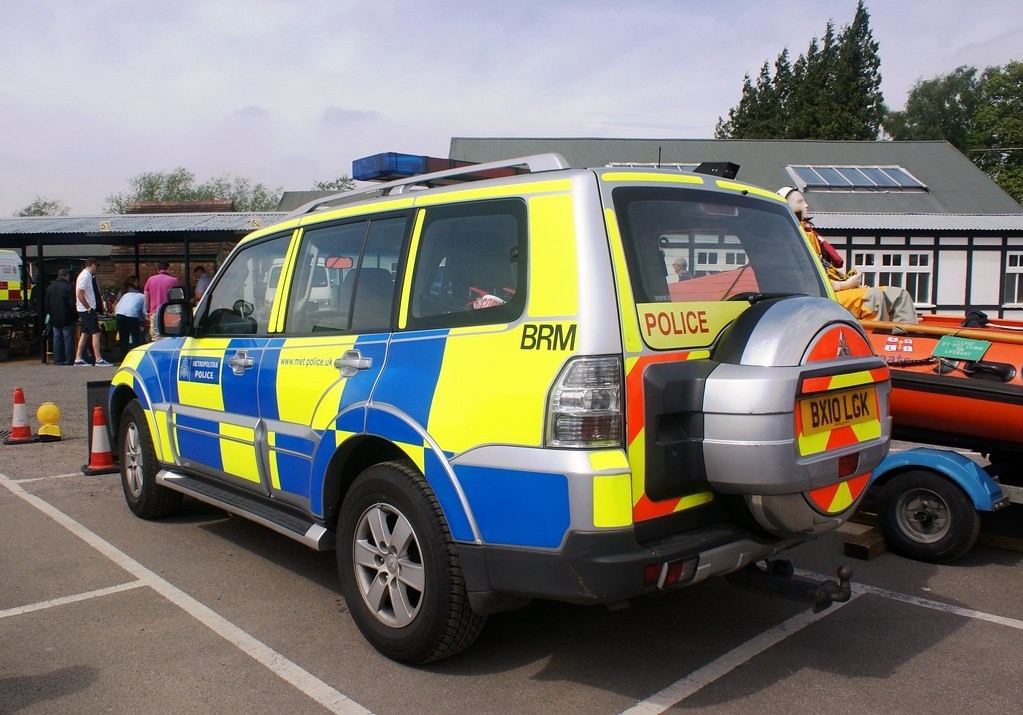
left=666, top=259, right=1023, bottom=566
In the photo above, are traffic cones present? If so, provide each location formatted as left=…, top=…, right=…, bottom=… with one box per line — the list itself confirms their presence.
left=3, top=386, right=39, bottom=445
left=80, top=405, right=121, bottom=476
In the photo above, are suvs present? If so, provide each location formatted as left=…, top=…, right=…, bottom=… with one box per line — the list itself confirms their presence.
left=109, top=149, right=894, bottom=671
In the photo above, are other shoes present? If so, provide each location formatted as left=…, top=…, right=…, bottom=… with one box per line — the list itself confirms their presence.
left=65, top=361, right=73, bottom=365
left=53, top=361, right=65, bottom=365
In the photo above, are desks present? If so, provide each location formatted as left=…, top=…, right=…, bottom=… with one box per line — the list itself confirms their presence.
left=76, top=318, right=116, bottom=363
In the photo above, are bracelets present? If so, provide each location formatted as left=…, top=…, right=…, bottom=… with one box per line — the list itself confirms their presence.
left=86, top=308, right=91, bottom=311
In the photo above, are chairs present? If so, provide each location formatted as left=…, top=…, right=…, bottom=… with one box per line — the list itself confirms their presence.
left=316, top=265, right=394, bottom=332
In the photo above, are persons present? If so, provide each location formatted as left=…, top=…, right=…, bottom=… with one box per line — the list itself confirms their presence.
left=191, top=265, right=211, bottom=307
left=114, top=291, right=147, bottom=362
left=673, top=257, right=694, bottom=282
left=116, top=276, right=140, bottom=305
left=73, top=258, right=114, bottom=367
left=44, top=269, right=77, bottom=365
left=778, top=186, right=916, bottom=323
left=29, top=272, right=52, bottom=363
left=144, top=261, right=178, bottom=341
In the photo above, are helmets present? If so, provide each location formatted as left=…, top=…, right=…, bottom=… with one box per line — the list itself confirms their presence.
left=775, top=185, right=799, bottom=198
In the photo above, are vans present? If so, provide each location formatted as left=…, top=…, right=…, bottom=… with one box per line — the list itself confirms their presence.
left=263, top=255, right=333, bottom=311
left=0, top=250, right=35, bottom=301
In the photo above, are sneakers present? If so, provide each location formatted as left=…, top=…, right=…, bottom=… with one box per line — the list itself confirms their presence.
left=95, top=359, right=114, bottom=367
left=74, top=359, right=93, bottom=367
